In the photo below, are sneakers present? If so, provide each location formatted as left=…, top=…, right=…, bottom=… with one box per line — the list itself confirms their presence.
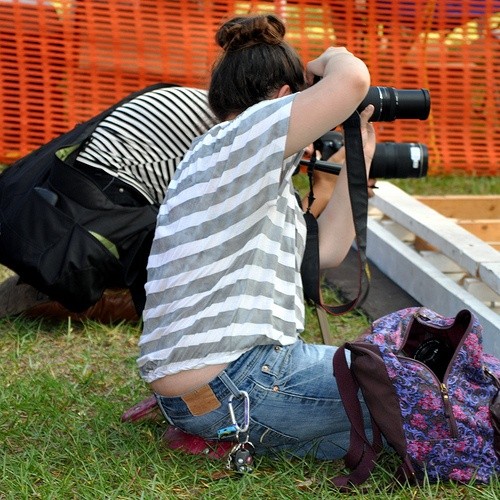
left=121, top=395, right=170, bottom=428
left=164, top=425, right=248, bottom=463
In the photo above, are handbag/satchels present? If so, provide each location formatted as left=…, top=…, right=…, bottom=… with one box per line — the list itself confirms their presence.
left=0, top=121, right=159, bottom=314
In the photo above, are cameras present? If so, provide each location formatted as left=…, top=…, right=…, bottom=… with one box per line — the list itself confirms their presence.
left=312, top=74, right=430, bottom=123
left=313, top=132, right=428, bottom=179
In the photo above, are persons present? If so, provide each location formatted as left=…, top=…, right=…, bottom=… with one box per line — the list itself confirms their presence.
left=0, top=14, right=394, bottom=470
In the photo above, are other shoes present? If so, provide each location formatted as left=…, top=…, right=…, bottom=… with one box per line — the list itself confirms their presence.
left=0, top=275, right=140, bottom=326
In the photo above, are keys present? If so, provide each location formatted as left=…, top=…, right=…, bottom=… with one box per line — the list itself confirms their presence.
left=217, top=389, right=256, bottom=473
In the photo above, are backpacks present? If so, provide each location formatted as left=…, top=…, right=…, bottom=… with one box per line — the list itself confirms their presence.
left=331, top=307, right=500, bottom=495
